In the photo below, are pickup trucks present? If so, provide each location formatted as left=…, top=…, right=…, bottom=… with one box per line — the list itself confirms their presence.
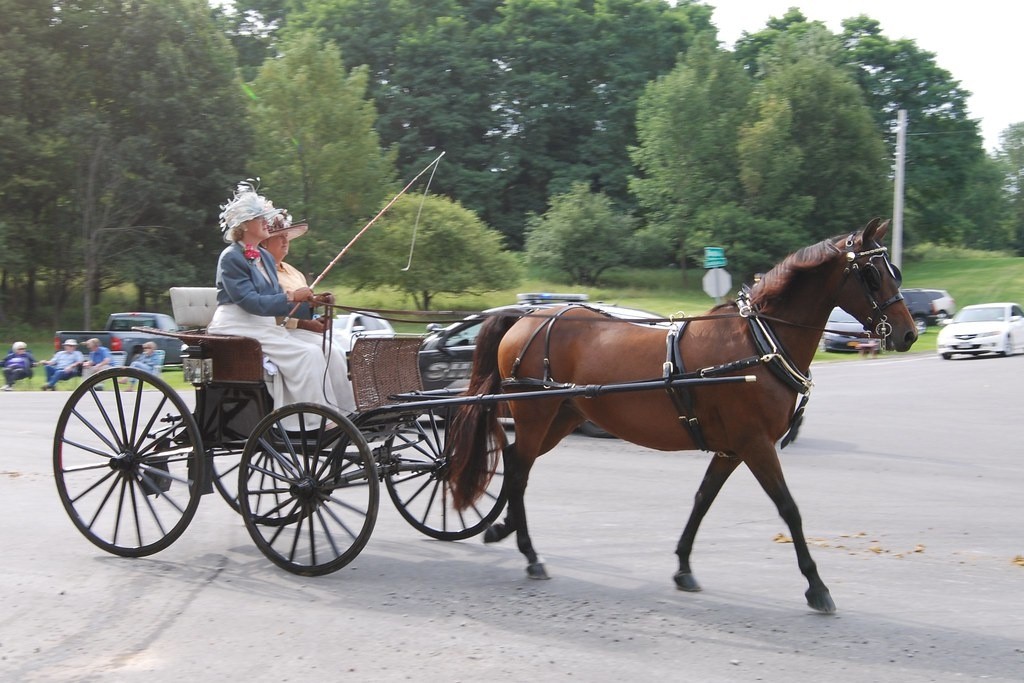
left=54, top=312, right=187, bottom=366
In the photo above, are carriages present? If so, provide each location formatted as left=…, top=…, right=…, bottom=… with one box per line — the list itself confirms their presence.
left=52, top=216, right=917, bottom=615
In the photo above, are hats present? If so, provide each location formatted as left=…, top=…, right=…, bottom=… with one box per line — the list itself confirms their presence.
left=217, top=176, right=286, bottom=244
left=61, top=338, right=79, bottom=347
left=263, top=209, right=309, bottom=242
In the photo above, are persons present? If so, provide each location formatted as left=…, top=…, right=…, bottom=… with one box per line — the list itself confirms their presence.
left=40, top=339, right=85, bottom=391
left=264, top=209, right=358, bottom=420
left=207, top=177, right=341, bottom=435
left=0, top=342, right=40, bottom=390
left=118, top=342, right=156, bottom=392
left=82, top=338, right=115, bottom=380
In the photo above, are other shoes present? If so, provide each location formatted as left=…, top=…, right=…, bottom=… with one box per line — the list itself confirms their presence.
left=41, top=382, right=54, bottom=389
left=124, top=384, right=137, bottom=391
left=1, top=382, right=13, bottom=391
left=116, top=377, right=127, bottom=384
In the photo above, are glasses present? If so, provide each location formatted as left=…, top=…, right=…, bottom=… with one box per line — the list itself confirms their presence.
left=17, top=347, right=26, bottom=350
left=142, top=346, right=151, bottom=349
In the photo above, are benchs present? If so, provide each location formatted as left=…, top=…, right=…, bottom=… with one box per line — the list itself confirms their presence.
left=169, top=286, right=264, bottom=386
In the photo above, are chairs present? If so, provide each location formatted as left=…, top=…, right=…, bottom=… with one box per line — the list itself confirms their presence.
left=108, top=350, right=127, bottom=390
left=138, top=349, right=166, bottom=389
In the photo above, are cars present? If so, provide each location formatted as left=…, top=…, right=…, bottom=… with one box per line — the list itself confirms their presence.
left=824, top=306, right=881, bottom=354
left=922, top=289, right=957, bottom=320
left=936, top=302, right=1024, bottom=360
left=417, top=292, right=674, bottom=438
left=898, top=289, right=937, bottom=336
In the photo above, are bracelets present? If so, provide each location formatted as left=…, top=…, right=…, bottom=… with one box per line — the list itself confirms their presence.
left=285, top=291, right=290, bottom=301
